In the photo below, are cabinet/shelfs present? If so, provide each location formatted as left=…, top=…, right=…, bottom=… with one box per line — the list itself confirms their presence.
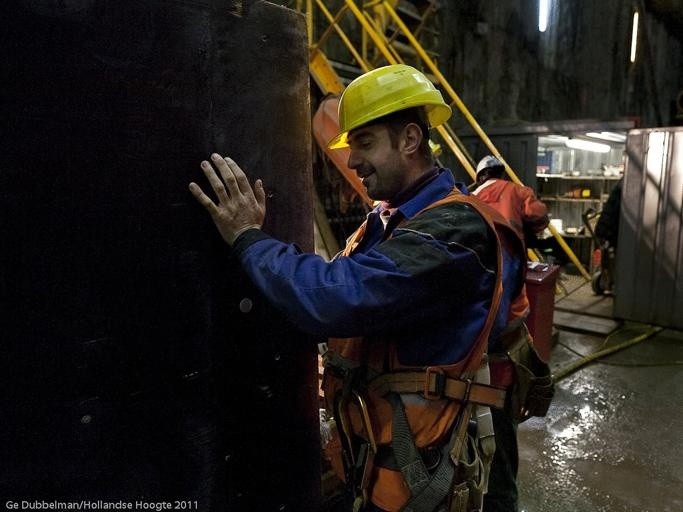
left=538, top=230, right=593, bottom=276
left=535, top=172, right=624, bottom=210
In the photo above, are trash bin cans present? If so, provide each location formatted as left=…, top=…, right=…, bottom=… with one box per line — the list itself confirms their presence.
left=526, top=261, right=560, bottom=361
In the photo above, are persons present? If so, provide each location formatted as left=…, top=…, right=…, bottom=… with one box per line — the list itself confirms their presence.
left=188, top=63, right=528, bottom=512
left=469, top=156, right=549, bottom=320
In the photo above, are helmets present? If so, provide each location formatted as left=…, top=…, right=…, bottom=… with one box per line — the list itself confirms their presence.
left=326, top=64, right=453, bottom=150
left=476, top=156, right=506, bottom=184
